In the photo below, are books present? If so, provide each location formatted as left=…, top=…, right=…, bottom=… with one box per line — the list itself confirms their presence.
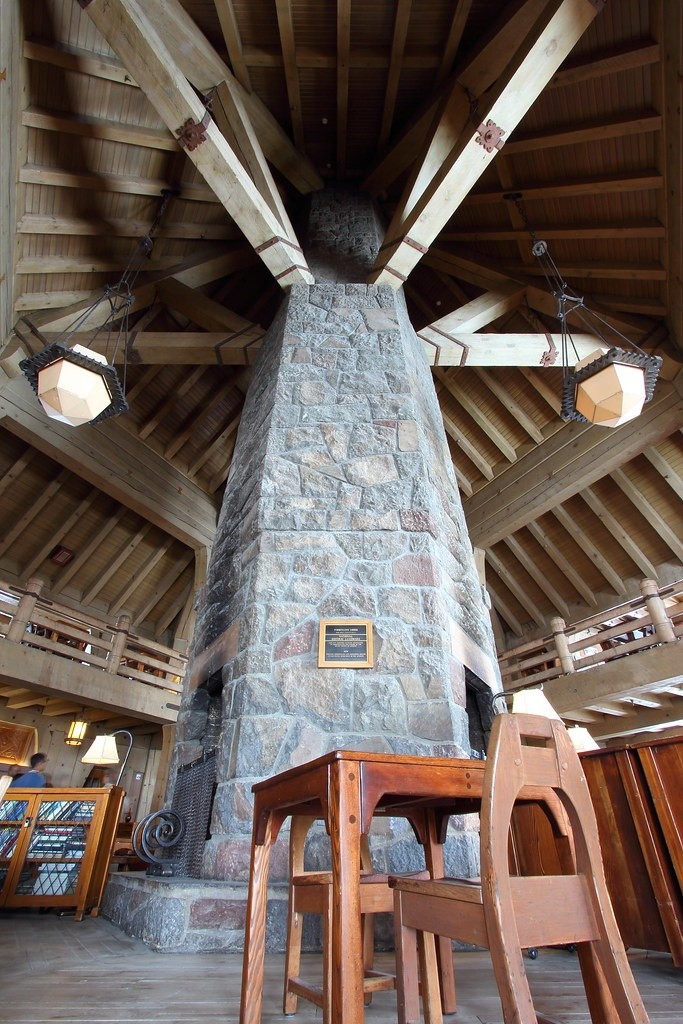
left=0, top=800, right=97, bottom=896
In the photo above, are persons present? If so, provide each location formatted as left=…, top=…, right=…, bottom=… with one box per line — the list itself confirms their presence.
left=8, top=764, right=20, bottom=780
left=10, top=753, right=48, bottom=787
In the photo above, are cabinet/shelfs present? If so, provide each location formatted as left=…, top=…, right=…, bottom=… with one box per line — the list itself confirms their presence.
left=0, top=784, right=127, bottom=921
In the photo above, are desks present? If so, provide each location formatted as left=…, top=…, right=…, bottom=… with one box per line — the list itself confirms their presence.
left=35, top=620, right=90, bottom=662
left=119, top=651, right=167, bottom=688
left=240, top=750, right=624, bottom=1024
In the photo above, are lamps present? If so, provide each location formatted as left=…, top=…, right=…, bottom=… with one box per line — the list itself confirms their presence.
left=17, top=188, right=176, bottom=428
left=64, top=707, right=91, bottom=746
left=567, top=725, right=601, bottom=753
left=501, top=191, right=663, bottom=428
left=490, top=688, right=565, bottom=725
left=80, top=730, right=133, bottom=787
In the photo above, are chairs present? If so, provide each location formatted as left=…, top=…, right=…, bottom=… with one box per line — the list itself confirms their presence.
left=388, top=712, right=653, bottom=1024
left=283, top=816, right=445, bottom=1024
